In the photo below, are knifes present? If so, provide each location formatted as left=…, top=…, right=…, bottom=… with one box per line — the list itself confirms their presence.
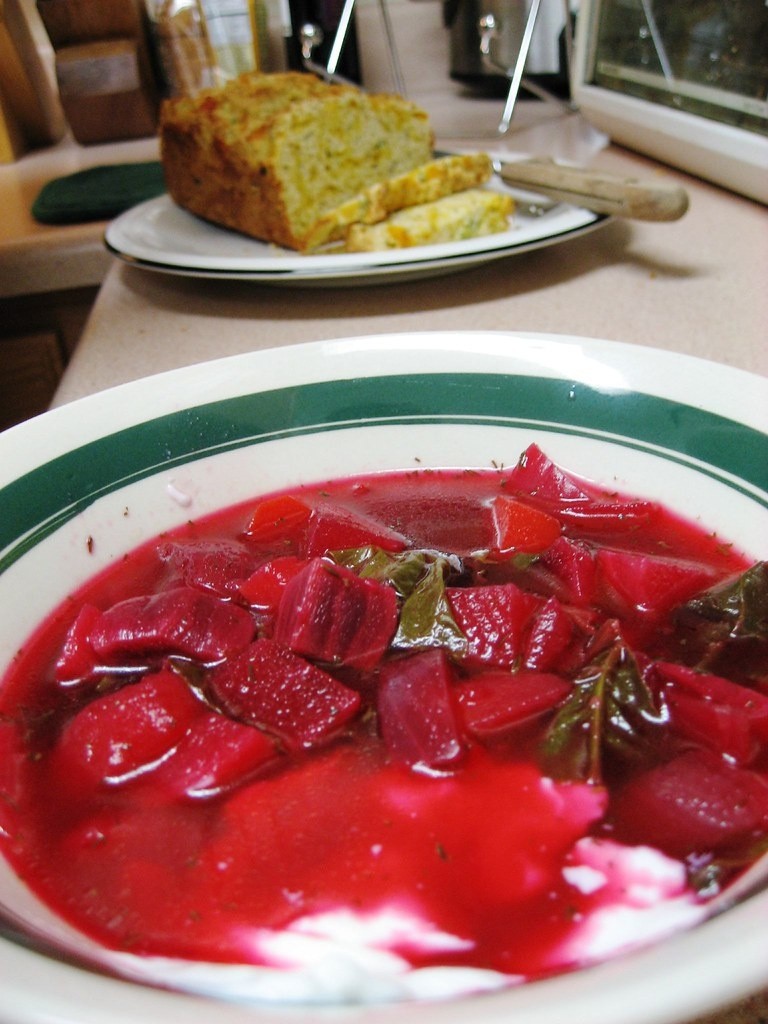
left=431, top=149, right=690, bottom=222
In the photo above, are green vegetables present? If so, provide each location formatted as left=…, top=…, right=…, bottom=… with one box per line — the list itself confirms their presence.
left=323, top=539, right=768, bottom=782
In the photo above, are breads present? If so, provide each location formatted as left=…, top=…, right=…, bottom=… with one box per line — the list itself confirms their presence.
left=158, top=71, right=517, bottom=251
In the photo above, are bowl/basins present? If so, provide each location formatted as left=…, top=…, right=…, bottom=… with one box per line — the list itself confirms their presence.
left=0, top=328, right=768, bottom=1024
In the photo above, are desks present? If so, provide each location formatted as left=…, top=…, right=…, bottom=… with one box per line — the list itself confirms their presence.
left=0, top=129, right=768, bottom=1024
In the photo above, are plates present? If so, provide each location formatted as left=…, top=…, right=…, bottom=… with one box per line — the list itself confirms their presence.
left=103, top=152, right=620, bottom=290
